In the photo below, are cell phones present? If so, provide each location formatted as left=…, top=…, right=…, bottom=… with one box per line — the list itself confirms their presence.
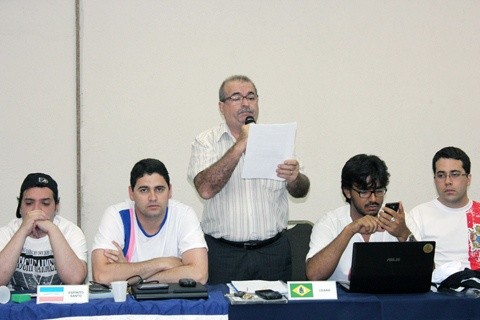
left=180, top=278, right=196, bottom=287
left=255, top=289, right=282, bottom=300
left=381, top=202, right=399, bottom=229
left=138, top=283, right=169, bottom=289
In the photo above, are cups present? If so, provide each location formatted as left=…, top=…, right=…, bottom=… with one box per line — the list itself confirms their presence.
left=110, top=281, right=128, bottom=302
left=0, top=285, right=10, bottom=304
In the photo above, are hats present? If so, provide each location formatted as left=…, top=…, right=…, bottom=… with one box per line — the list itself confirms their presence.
left=16, top=173, right=58, bottom=219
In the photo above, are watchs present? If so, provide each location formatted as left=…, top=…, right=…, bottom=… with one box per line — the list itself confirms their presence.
left=405, top=232, right=416, bottom=242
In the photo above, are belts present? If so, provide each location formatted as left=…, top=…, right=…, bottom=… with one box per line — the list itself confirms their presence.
left=211, top=233, right=281, bottom=250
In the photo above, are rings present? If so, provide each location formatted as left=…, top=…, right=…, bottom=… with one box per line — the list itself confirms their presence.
left=290, top=170, right=293, bottom=175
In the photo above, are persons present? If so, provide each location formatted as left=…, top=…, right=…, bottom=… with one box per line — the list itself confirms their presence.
left=406, top=146, right=480, bottom=290
left=305, top=154, right=420, bottom=282
left=91, top=159, right=210, bottom=285
left=0, top=173, right=89, bottom=291
left=186, top=74, right=310, bottom=285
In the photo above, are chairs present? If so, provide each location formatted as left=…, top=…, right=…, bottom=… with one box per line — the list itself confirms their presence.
left=287, top=221, right=315, bottom=281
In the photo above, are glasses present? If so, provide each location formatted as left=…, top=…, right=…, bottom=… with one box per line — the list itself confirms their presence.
left=88, top=280, right=112, bottom=292
left=220, top=91, right=259, bottom=105
left=126, top=275, right=160, bottom=288
left=434, top=170, right=467, bottom=180
left=350, top=186, right=387, bottom=198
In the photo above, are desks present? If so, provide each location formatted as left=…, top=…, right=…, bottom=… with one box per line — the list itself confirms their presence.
left=0, top=283, right=480, bottom=320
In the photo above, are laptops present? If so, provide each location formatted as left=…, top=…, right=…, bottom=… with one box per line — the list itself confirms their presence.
left=132, top=282, right=208, bottom=300
left=336, top=240, right=436, bottom=292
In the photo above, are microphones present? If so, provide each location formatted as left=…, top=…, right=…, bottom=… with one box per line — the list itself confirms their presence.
left=245, top=116, right=254, bottom=125
